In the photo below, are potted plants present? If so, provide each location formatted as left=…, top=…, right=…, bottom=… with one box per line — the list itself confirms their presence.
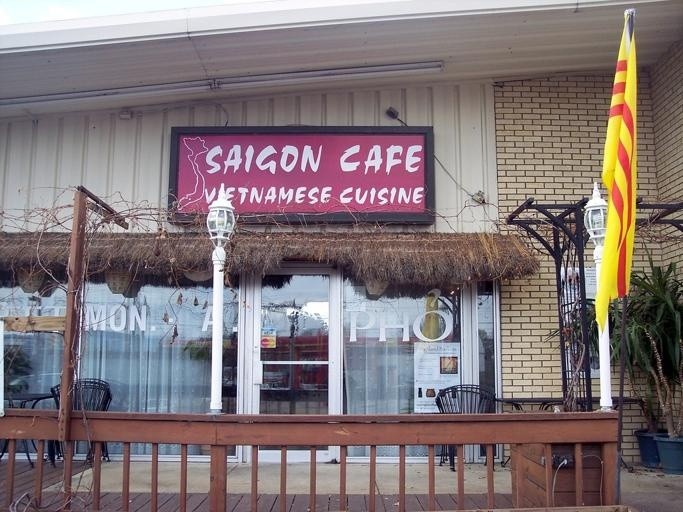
left=543, top=241, right=683, bottom=475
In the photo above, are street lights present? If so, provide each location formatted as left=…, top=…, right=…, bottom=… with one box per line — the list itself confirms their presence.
left=581, top=181, right=616, bottom=413
left=287, top=295, right=298, bottom=390
left=4, top=372, right=61, bottom=398
left=202, top=181, right=239, bottom=415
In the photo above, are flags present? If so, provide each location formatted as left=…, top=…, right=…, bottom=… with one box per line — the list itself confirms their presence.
left=591, top=8, right=644, bottom=338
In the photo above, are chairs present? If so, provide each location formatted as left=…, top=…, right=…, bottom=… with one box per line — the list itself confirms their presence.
left=44, top=377, right=113, bottom=468
left=435, top=383, right=503, bottom=472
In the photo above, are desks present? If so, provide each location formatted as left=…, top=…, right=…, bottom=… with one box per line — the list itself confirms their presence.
left=0, top=392, right=59, bottom=469
left=495, top=395, right=639, bottom=473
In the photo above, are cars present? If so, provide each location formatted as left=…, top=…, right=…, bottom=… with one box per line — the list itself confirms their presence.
left=220, top=362, right=287, bottom=389
